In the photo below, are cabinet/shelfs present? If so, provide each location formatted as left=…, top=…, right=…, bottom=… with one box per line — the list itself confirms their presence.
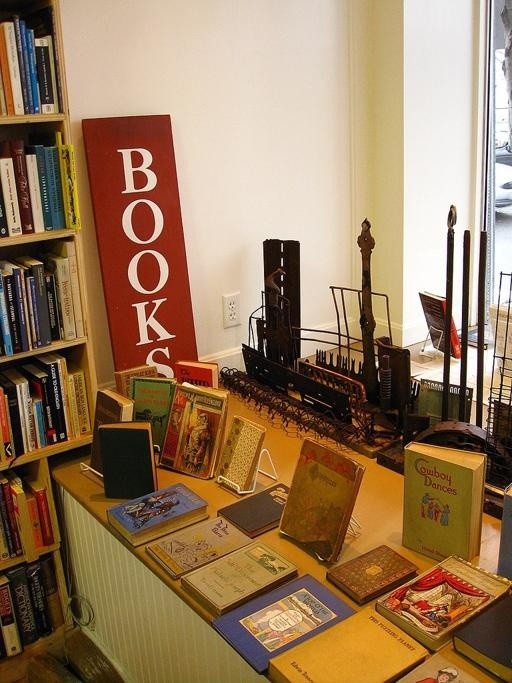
left=0, top=1, right=99, bottom=666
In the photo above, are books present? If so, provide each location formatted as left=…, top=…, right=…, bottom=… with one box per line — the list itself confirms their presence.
left=1, top=17, right=512, bottom=683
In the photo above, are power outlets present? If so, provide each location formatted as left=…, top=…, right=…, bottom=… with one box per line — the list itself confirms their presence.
left=222, top=292, right=242, bottom=328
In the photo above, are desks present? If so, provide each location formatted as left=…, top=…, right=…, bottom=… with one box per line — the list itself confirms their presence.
left=51, top=383, right=512, bottom=683
